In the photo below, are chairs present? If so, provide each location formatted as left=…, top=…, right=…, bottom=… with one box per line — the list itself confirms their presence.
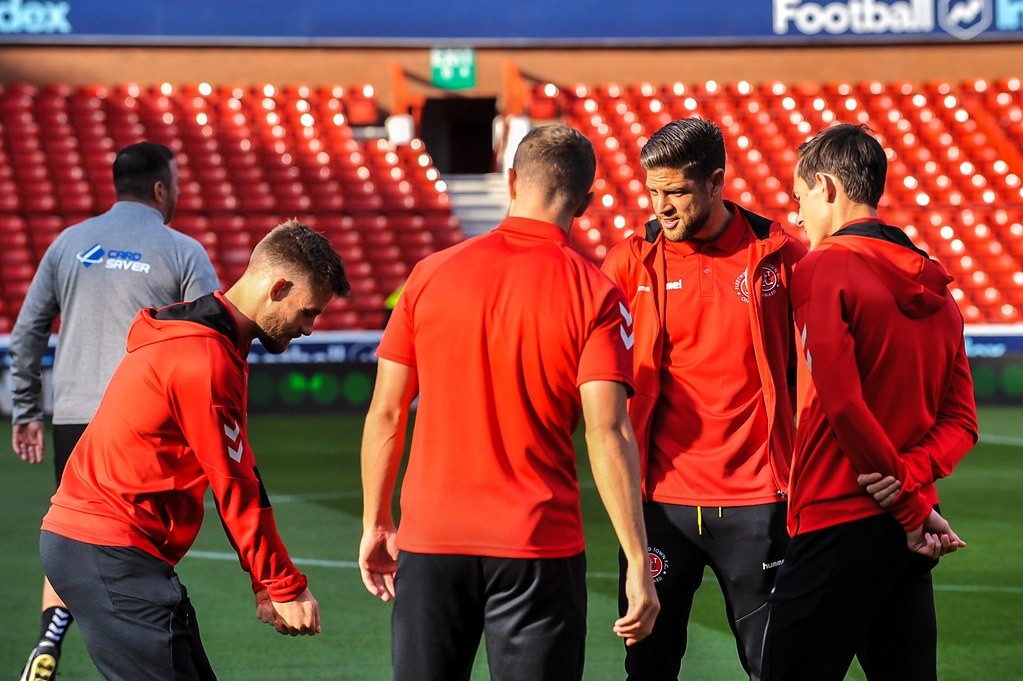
left=0, top=84, right=467, bottom=331
left=530, top=79, right=1023, bottom=326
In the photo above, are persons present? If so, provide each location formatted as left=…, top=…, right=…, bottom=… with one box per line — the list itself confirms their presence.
left=358, top=127, right=661, bottom=681
left=10, top=141, right=222, bottom=681
left=38, top=218, right=351, bottom=681
left=601, top=118, right=809, bottom=681
left=763, top=122, right=979, bottom=681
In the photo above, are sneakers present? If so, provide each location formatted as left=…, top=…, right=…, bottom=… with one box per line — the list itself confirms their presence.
left=19, top=638, right=60, bottom=681
